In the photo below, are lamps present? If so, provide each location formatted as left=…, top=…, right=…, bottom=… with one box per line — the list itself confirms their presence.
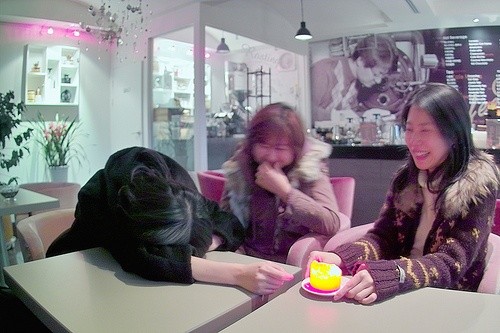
left=217, top=13, right=231, bottom=52
left=294, top=0, right=312, bottom=40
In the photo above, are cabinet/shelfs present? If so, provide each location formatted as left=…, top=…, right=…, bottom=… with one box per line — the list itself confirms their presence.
left=23, top=43, right=82, bottom=105
left=247, top=66, right=272, bottom=122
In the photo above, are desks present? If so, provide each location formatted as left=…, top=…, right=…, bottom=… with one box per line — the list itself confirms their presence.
left=0, top=250, right=500, bottom=333
left=0, top=183, right=80, bottom=247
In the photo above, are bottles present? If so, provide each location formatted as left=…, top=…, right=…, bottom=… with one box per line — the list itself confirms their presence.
left=27, top=89, right=35, bottom=102
left=61, top=74, right=70, bottom=83
left=61, top=89, right=71, bottom=102
left=345, top=114, right=383, bottom=145
left=33, top=63, right=39, bottom=71
left=47, top=68, right=54, bottom=88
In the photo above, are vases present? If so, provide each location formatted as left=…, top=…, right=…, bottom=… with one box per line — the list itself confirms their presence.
left=47, top=163, right=69, bottom=184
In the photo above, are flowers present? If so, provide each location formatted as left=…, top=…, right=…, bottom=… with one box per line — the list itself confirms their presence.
left=26, top=110, right=89, bottom=171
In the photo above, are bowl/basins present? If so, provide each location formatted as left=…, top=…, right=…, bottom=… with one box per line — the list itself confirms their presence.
left=0, top=185, right=20, bottom=199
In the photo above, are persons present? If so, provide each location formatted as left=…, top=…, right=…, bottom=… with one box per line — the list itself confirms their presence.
left=305, top=82, right=500, bottom=305
left=46, top=147, right=294, bottom=295
left=310, top=34, right=399, bottom=128
left=218, top=102, right=341, bottom=263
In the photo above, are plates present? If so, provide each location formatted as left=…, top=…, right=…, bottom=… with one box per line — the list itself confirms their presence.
left=301, top=276, right=351, bottom=296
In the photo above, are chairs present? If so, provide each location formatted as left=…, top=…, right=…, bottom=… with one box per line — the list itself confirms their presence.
left=325, top=222, right=500, bottom=292
left=195, top=170, right=356, bottom=265
left=16, top=209, right=80, bottom=261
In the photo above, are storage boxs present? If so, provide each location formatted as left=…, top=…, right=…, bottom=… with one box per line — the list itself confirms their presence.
left=154, top=108, right=184, bottom=122
